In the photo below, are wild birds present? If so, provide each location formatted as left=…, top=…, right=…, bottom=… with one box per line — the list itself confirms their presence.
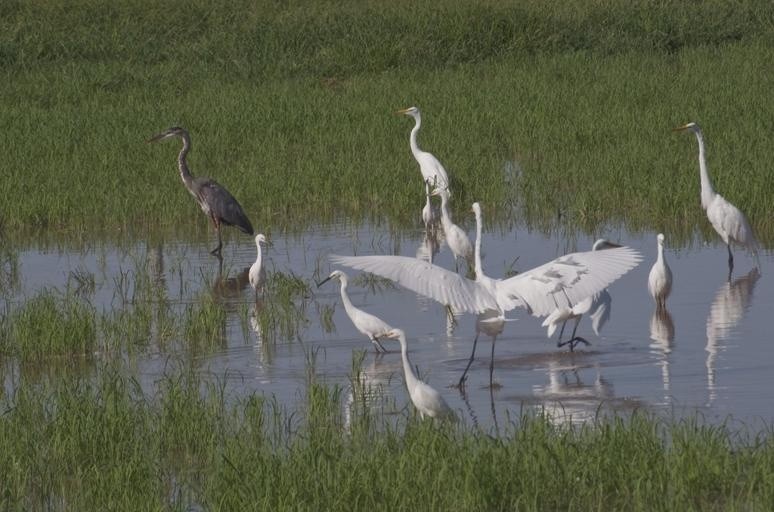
left=248, top=233, right=273, bottom=298
left=649, top=304, right=674, bottom=391
left=148, top=127, right=253, bottom=259
left=317, top=270, right=392, bottom=354
left=370, top=328, right=458, bottom=423
left=327, top=202, right=644, bottom=387
left=671, top=123, right=756, bottom=262
left=396, top=106, right=474, bottom=274
left=694, top=263, right=762, bottom=410
left=647, top=233, right=672, bottom=304
left=541, top=239, right=622, bottom=352
